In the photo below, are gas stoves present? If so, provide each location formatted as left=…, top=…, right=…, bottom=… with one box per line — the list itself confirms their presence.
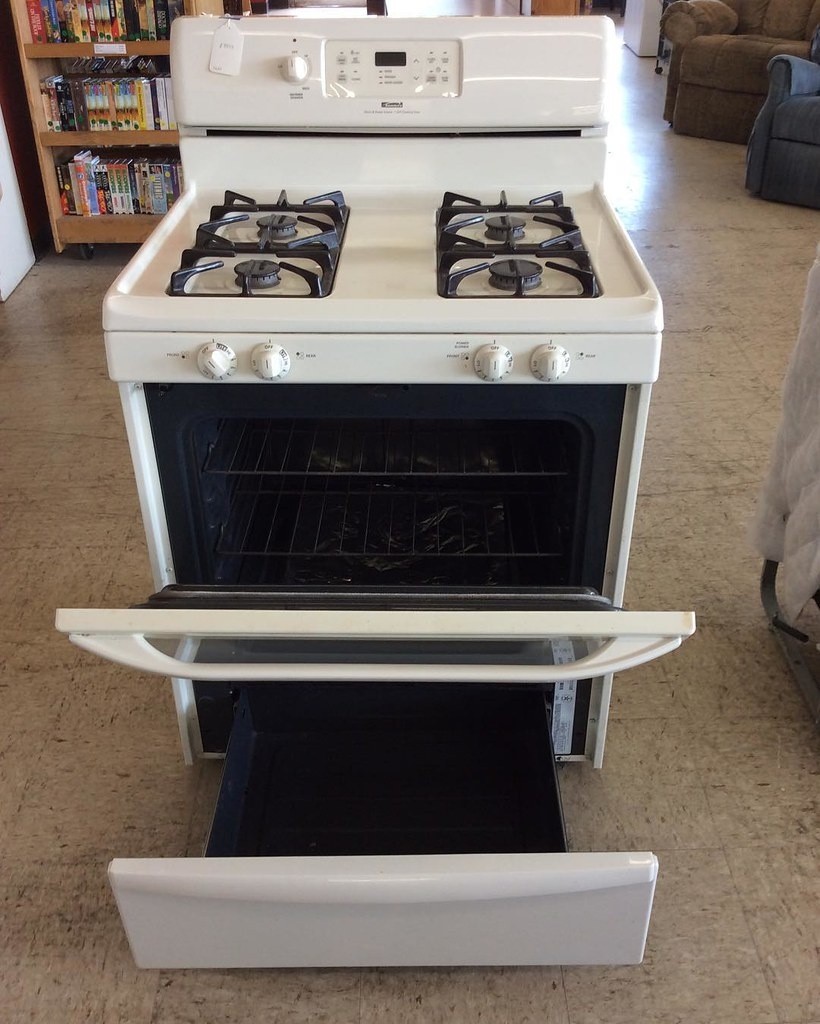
left=100, top=15, right=666, bottom=334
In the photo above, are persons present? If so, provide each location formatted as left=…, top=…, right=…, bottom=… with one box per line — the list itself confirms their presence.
left=156, top=166, right=161, bottom=174
left=149, top=165, right=155, bottom=173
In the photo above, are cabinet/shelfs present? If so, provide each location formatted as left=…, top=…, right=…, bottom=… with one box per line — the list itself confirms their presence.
left=7, top=0, right=226, bottom=261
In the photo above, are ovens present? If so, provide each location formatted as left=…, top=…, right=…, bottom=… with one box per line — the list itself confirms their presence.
left=53, top=330, right=696, bottom=969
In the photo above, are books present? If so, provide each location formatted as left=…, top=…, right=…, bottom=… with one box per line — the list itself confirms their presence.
left=65, top=57, right=157, bottom=77
left=55, top=149, right=183, bottom=215
left=26, top=0, right=185, bottom=43
left=223, top=0, right=289, bottom=16
left=38, top=72, right=178, bottom=132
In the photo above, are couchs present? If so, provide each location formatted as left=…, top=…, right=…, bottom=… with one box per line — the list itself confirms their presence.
left=744, top=26, right=820, bottom=210
left=659, top=0, right=820, bottom=146
